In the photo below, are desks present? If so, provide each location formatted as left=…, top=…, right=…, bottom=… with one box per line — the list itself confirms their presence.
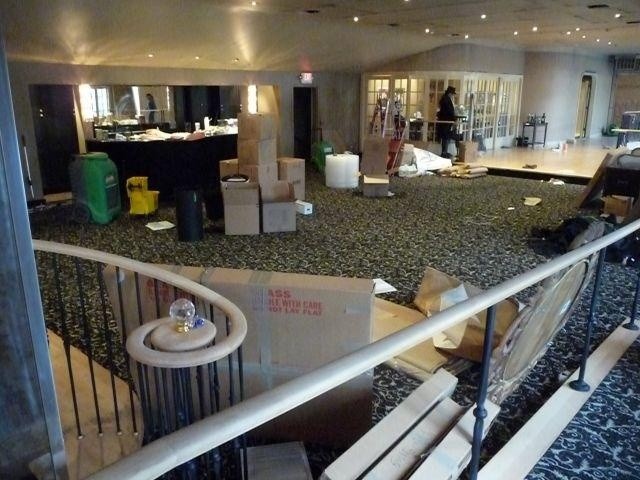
left=521, top=122, right=548, bottom=149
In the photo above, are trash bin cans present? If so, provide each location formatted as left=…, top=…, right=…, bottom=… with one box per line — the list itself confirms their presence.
left=459, top=141, right=474, bottom=162
left=176, top=189, right=202, bottom=241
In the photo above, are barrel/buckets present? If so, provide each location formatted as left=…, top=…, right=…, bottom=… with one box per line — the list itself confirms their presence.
left=325, top=153, right=359, bottom=187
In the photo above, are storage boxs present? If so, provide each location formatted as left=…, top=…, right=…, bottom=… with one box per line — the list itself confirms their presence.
left=102, top=262, right=375, bottom=449
left=459, top=141, right=478, bottom=163
left=218, top=112, right=305, bottom=237
left=363, top=173, right=390, bottom=197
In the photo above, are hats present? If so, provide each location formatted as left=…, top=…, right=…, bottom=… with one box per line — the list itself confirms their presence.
left=445, top=85, right=456, bottom=94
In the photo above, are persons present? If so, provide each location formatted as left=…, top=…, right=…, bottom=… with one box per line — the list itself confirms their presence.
left=437, top=86, right=458, bottom=158
left=145, top=92, right=157, bottom=124
